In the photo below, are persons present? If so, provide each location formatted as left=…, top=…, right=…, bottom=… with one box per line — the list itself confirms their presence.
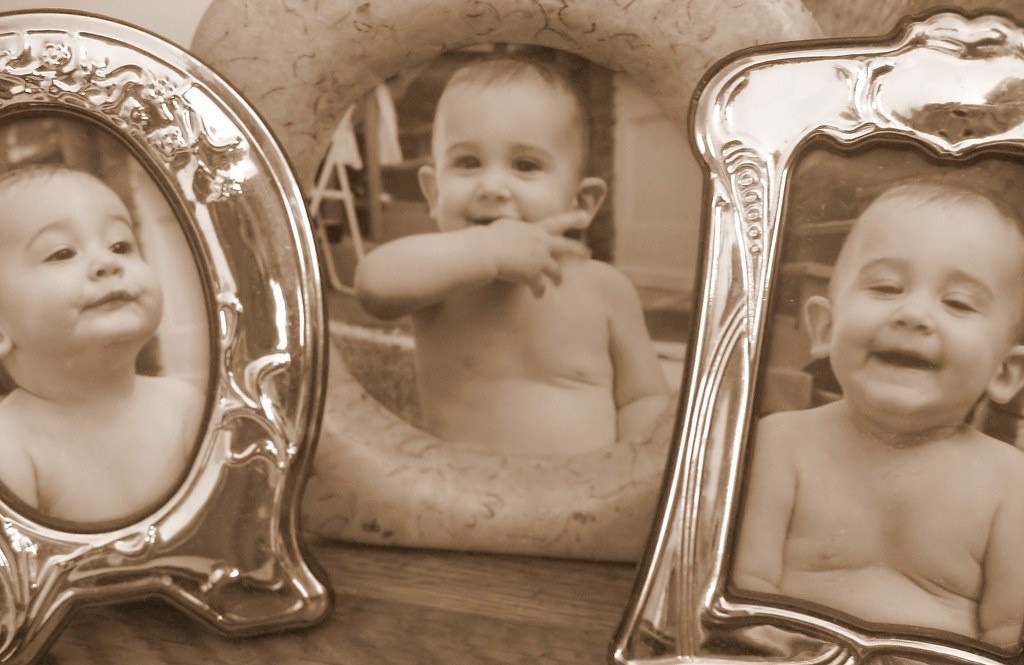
left=351, top=59, right=671, bottom=450
left=731, top=181, right=1024, bottom=659
left=2, top=162, right=211, bottom=530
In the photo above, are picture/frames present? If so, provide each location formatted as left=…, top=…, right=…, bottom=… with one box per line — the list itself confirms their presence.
left=0, top=0, right=1024, bottom=665
